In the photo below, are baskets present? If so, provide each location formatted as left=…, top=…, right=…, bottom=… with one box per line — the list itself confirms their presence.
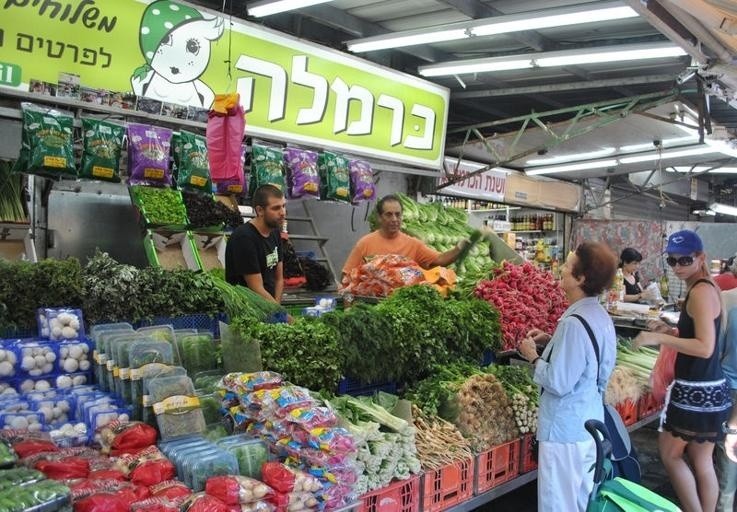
left=134, top=310, right=287, bottom=339
left=334, top=432, right=539, bottom=511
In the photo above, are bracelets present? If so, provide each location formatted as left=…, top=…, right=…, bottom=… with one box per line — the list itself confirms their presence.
left=721, top=419, right=736, bottom=434
left=531, top=355, right=542, bottom=366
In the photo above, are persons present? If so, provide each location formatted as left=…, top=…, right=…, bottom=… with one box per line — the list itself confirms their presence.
left=720, top=403, right=736, bottom=464
left=224, top=183, right=296, bottom=325
left=619, top=247, right=655, bottom=303
left=631, top=230, right=731, bottom=511
left=712, top=256, right=736, bottom=291
left=339, top=194, right=472, bottom=289
left=710, top=306, right=736, bottom=512
left=516, top=242, right=619, bottom=512
left=718, top=253, right=736, bottom=310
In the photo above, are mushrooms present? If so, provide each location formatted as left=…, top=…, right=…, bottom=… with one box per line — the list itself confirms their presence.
left=0, top=308, right=127, bottom=447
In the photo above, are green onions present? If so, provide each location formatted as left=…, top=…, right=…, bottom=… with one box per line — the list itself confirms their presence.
left=0, top=158, right=31, bottom=224
left=616, top=333, right=660, bottom=384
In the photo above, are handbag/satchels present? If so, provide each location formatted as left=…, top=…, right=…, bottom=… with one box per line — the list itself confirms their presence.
left=603, top=402, right=644, bottom=483
left=651, top=327, right=680, bottom=402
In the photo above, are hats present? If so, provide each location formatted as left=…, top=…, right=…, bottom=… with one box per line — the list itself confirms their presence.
left=660, top=229, right=704, bottom=257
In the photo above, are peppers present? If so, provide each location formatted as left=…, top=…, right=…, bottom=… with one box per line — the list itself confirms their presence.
left=0, top=441, right=71, bottom=512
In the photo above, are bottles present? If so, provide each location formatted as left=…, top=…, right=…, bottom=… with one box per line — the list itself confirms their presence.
left=443, top=194, right=556, bottom=232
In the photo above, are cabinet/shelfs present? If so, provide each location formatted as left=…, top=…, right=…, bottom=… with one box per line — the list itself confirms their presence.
left=433, top=194, right=559, bottom=260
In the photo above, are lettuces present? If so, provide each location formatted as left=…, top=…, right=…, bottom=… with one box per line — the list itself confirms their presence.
left=367, top=192, right=491, bottom=283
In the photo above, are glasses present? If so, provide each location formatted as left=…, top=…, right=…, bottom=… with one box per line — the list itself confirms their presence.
left=667, top=256, right=694, bottom=267
